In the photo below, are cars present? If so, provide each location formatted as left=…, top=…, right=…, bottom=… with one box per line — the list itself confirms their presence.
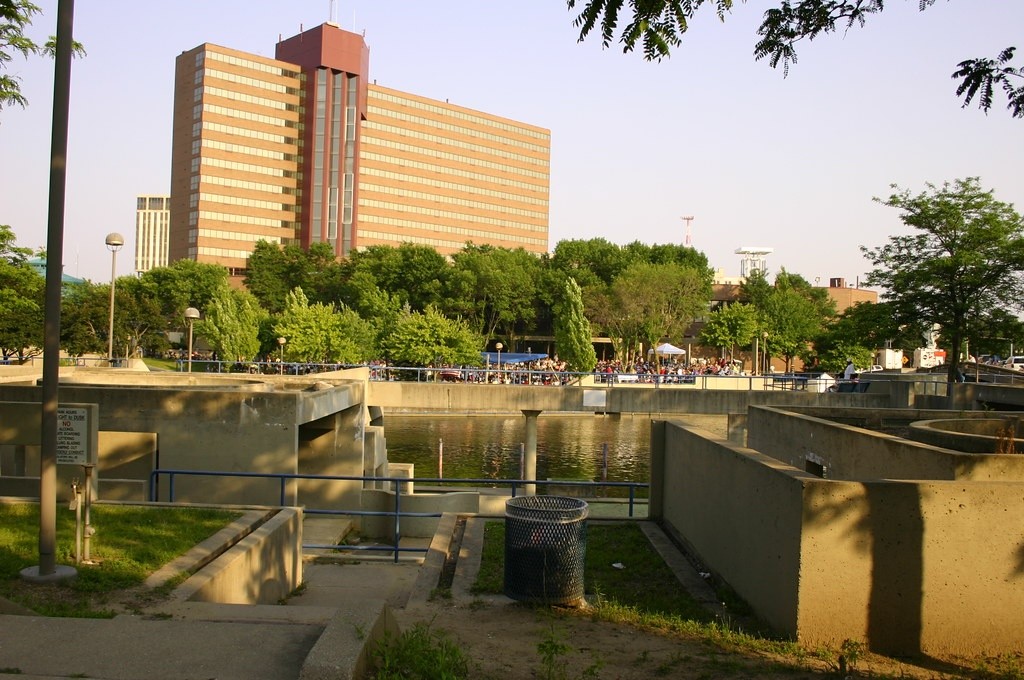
left=1002, top=356, right=1024, bottom=372
left=867, top=365, right=884, bottom=371
left=978, top=354, right=1007, bottom=368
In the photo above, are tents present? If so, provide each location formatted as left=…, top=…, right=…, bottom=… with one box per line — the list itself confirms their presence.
left=649, top=343, right=686, bottom=367
left=480, top=352, right=549, bottom=364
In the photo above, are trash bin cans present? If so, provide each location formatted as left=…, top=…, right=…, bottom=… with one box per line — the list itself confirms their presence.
left=503, top=494, right=590, bottom=605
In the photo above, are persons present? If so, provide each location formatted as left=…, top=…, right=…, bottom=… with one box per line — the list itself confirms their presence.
left=835, top=358, right=856, bottom=381
left=427, top=356, right=744, bottom=386
left=144, top=347, right=396, bottom=382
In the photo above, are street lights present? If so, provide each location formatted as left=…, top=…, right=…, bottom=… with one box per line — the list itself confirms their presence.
left=495, top=342, right=503, bottom=383
left=105, top=232, right=124, bottom=364
left=186, top=307, right=201, bottom=371
left=277, top=337, right=286, bottom=375
left=761, top=333, right=769, bottom=375
